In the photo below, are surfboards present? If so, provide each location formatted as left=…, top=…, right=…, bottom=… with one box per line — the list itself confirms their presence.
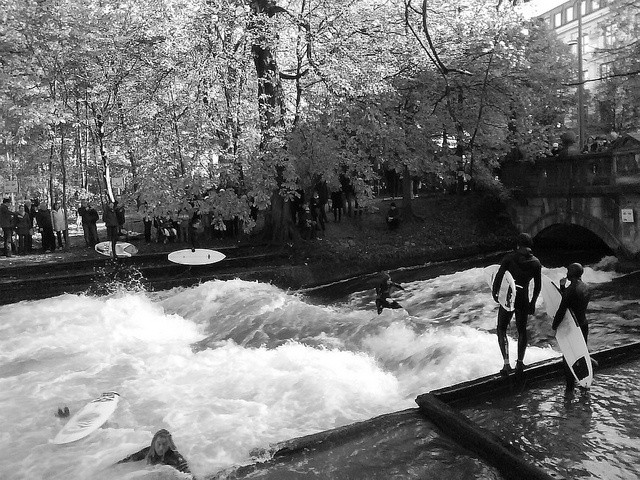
left=168, top=249, right=225, bottom=265
left=483, top=265, right=517, bottom=312
left=541, top=274, right=593, bottom=390
left=54, top=391, right=120, bottom=444
left=94, top=241, right=138, bottom=258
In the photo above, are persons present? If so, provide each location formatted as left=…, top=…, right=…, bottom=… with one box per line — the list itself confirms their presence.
left=50, top=199, right=68, bottom=250
left=385, top=202, right=400, bottom=231
left=14, top=204, right=31, bottom=256
left=374, top=271, right=412, bottom=315
left=142, top=169, right=372, bottom=244
left=78, top=198, right=99, bottom=247
left=582, top=131, right=621, bottom=155
left=1, top=198, right=12, bottom=256
left=550, top=142, right=561, bottom=158
left=13, top=199, right=36, bottom=253
left=119, top=206, right=126, bottom=235
left=491, top=230, right=541, bottom=374
left=36, top=202, right=56, bottom=253
left=112, top=428, right=197, bottom=480
left=102, top=202, right=118, bottom=241
left=551, top=262, right=596, bottom=396
